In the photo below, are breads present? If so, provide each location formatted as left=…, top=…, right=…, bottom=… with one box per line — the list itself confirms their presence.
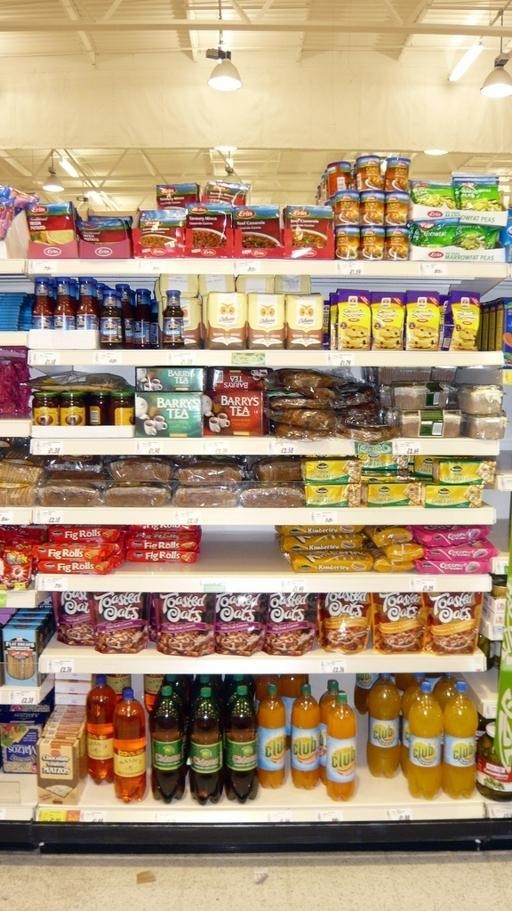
left=40, top=455, right=305, bottom=508
left=270, top=369, right=398, bottom=441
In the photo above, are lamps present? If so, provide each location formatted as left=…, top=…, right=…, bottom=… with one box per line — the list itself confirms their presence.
left=42, top=150, right=64, bottom=192
left=208, top=0, right=241, bottom=91
left=480, top=9, right=512, bottom=99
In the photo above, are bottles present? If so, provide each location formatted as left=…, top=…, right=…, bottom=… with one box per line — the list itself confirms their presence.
left=477, top=557, right=506, bottom=672
left=32, top=278, right=185, bottom=350
left=88, top=673, right=512, bottom=803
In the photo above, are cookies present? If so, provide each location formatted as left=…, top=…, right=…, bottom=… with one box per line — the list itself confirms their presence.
left=1, top=448, right=44, bottom=507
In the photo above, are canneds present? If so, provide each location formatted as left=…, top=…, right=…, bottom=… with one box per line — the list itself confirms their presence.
left=31, top=391, right=134, bottom=425
left=319, top=155, right=410, bottom=260
left=477, top=721, right=512, bottom=801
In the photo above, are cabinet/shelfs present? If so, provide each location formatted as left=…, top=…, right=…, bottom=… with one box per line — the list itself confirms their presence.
left=0, top=259, right=512, bottom=854
